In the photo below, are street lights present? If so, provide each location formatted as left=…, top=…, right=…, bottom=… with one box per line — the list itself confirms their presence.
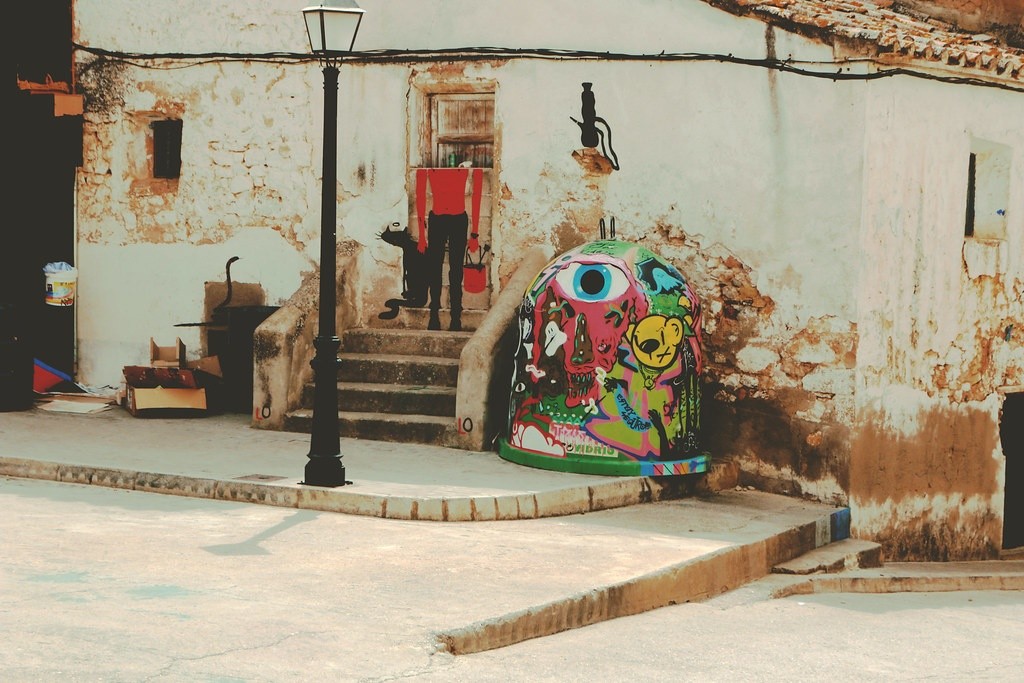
left=303, top=1, right=366, bottom=488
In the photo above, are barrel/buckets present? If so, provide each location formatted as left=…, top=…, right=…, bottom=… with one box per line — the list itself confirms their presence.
left=44, top=267, right=78, bottom=306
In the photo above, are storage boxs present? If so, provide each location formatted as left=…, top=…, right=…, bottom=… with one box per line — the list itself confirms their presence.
left=121, top=364, right=208, bottom=418
left=150, top=337, right=186, bottom=369
